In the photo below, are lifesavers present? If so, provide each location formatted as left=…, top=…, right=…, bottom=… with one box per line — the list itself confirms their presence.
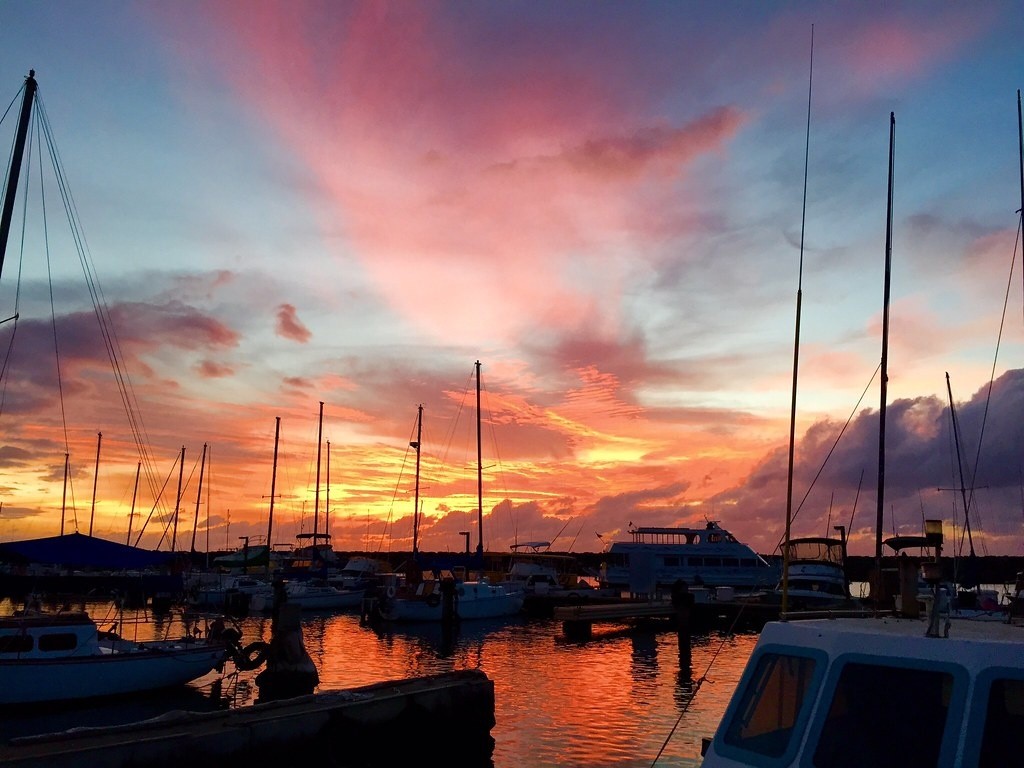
left=387, top=585, right=396, bottom=598
left=236, top=641, right=270, bottom=672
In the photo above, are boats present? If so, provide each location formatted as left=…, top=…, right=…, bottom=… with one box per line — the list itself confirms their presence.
left=774, top=468, right=864, bottom=611
left=596, top=520, right=776, bottom=593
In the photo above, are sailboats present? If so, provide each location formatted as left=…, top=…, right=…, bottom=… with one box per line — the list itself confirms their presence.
left=0, top=360, right=569, bottom=628
left=698, top=23, right=1023, bottom=767
left=0, top=69, right=241, bottom=706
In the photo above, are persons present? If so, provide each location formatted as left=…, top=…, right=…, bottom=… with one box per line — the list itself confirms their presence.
left=477, top=575, right=488, bottom=584
left=259, top=606, right=319, bottom=704
left=24, top=594, right=41, bottom=616
